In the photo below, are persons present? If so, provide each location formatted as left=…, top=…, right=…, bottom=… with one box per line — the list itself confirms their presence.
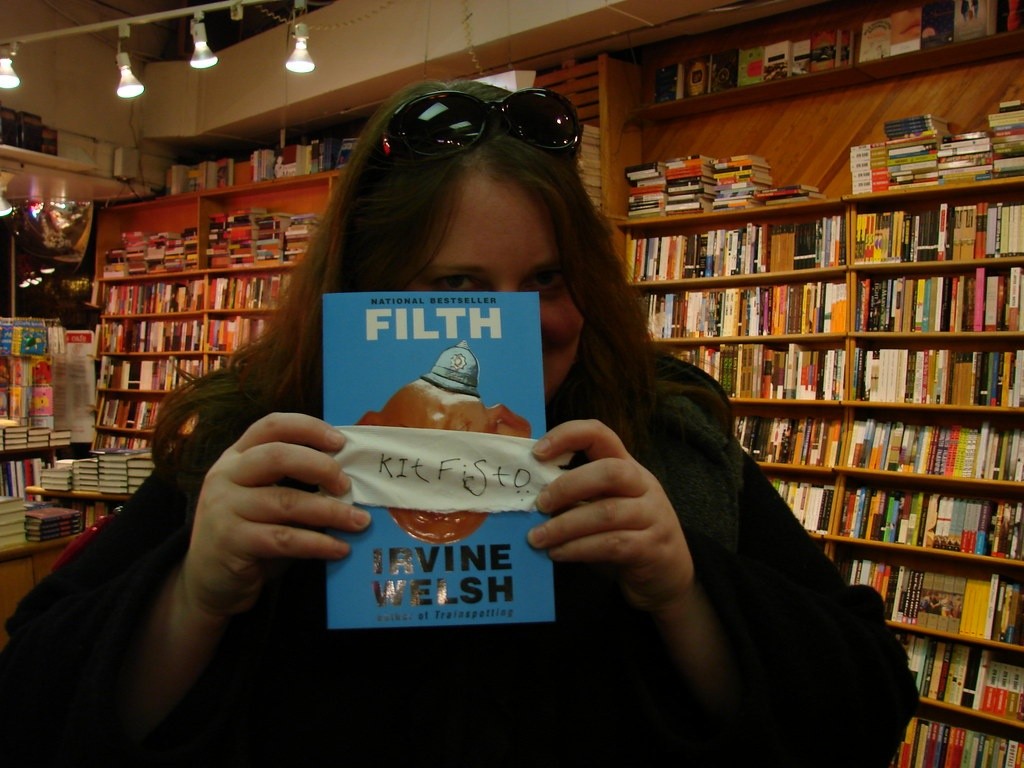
left=0, top=78, right=916, bottom=768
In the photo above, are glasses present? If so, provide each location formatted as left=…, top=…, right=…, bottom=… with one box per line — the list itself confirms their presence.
left=385, top=82, right=586, bottom=155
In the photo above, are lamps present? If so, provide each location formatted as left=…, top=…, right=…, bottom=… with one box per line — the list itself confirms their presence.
left=285, top=0, right=315, bottom=73
left=190, top=11, right=218, bottom=69
left=0, top=42, right=20, bottom=89
left=115, top=25, right=145, bottom=99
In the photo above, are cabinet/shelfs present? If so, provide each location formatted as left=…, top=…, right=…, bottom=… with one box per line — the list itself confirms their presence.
left=0, top=0, right=1023, bottom=768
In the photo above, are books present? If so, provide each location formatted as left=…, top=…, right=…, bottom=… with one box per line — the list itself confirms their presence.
left=888, top=717, right=1024, bottom=768
left=833, top=553, right=1024, bottom=645
left=769, top=475, right=1024, bottom=562
left=855, top=200, right=1024, bottom=331
left=889, top=629, right=1024, bottom=722
left=207, top=207, right=320, bottom=268
left=102, top=226, right=200, bottom=279
left=624, top=99, right=1024, bottom=218
left=0, top=419, right=155, bottom=551
left=207, top=273, right=295, bottom=375
left=669, top=343, right=1024, bottom=410
left=575, top=122, right=603, bottom=215
left=731, top=413, right=1024, bottom=483
left=162, top=137, right=360, bottom=197
left=0, top=103, right=59, bottom=157
left=647, top=0, right=1024, bottom=102
left=96, top=284, right=204, bottom=449
left=625, top=215, right=846, bottom=338
left=321, top=290, right=557, bottom=628
